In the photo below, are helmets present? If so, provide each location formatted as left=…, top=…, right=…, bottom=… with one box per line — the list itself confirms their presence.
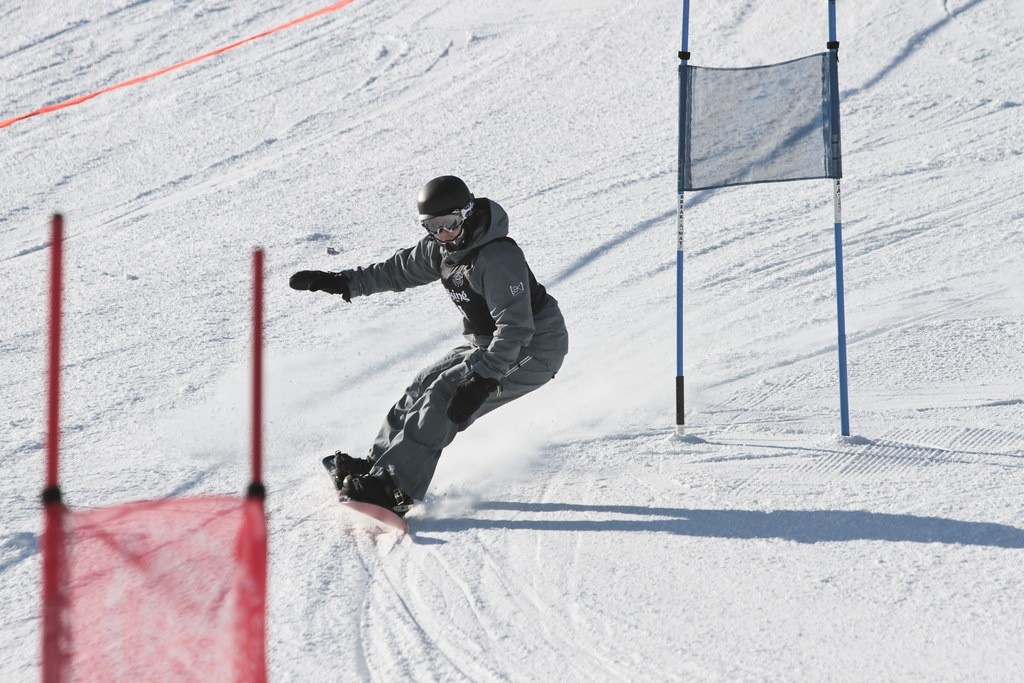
left=416, top=175, right=475, bottom=220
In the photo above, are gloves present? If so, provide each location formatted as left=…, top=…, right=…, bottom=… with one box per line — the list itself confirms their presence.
left=289, top=270, right=336, bottom=293
left=447, top=370, right=503, bottom=425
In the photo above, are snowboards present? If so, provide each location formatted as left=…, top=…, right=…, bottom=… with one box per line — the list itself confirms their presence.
left=321, top=454, right=409, bottom=540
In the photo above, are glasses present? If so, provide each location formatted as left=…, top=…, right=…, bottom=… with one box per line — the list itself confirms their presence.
left=421, top=214, right=464, bottom=235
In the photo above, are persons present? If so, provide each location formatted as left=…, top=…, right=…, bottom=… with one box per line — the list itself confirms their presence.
left=290, top=175, right=570, bottom=517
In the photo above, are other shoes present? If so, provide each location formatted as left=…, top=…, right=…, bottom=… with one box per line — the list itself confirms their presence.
left=335, top=451, right=377, bottom=478
left=344, top=469, right=414, bottom=516
